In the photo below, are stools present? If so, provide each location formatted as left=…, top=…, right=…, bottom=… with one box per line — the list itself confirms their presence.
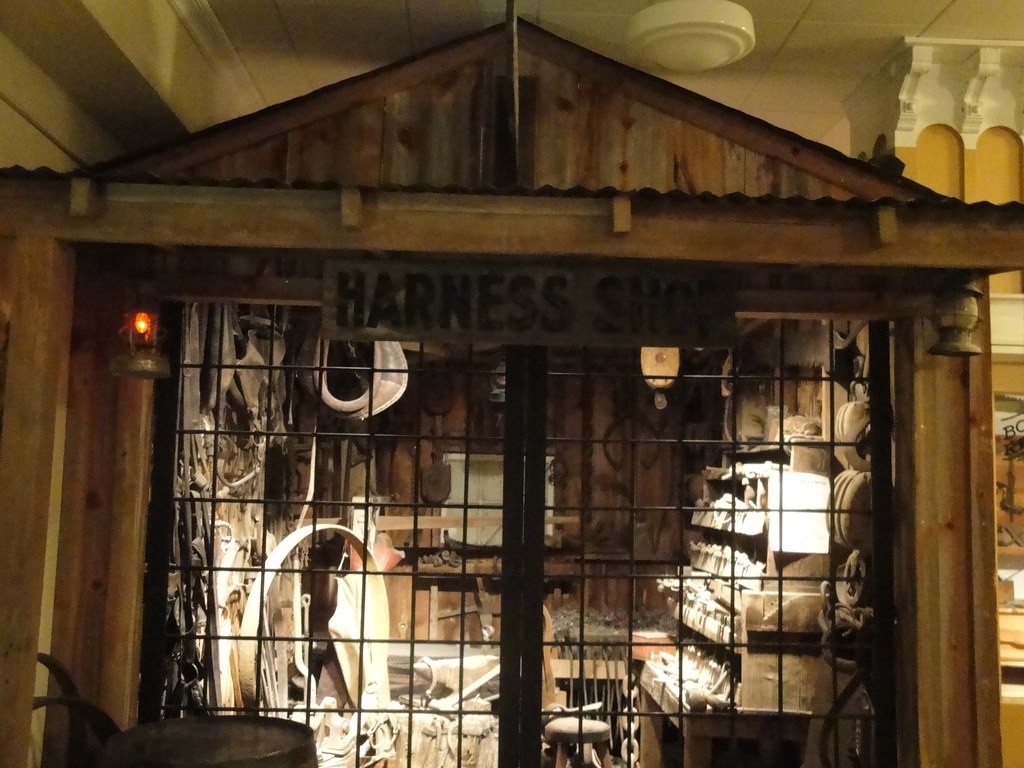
left=546, top=717, right=614, bottom=768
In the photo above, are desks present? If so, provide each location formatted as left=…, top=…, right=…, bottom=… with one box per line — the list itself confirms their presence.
left=637, top=662, right=836, bottom=768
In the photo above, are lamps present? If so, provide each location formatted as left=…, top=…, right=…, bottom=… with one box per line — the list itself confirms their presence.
left=640, top=348, right=680, bottom=410
left=117, top=297, right=168, bottom=376
left=925, top=273, right=985, bottom=359
left=629, top=0, right=756, bottom=72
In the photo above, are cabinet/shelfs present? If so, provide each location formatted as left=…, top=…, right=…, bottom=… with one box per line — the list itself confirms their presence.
left=667, top=436, right=868, bottom=714
